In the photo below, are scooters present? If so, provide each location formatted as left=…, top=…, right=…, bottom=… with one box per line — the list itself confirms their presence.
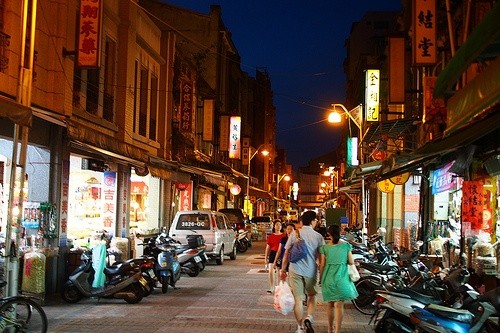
left=341, top=225, right=500, bottom=333
left=61, top=230, right=209, bottom=304
left=235, top=229, right=249, bottom=252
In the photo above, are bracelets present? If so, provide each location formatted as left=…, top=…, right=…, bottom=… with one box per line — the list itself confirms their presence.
left=274, top=258, right=278, bottom=264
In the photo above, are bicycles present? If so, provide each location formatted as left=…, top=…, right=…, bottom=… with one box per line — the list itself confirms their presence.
left=0, top=279, right=47, bottom=333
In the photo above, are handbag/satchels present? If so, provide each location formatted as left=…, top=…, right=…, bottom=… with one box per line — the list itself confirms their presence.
left=288, top=229, right=308, bottom=263
left=272, top=267, right=278, bottom=285
left=347, top=263, right=360, bottom=282
left=273, top=280, right=295, bottom=316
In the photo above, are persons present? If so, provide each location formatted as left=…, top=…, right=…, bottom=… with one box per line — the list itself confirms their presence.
left=273, top=224, right=297, bottom=286
left=319, top=224, right=359, bottom=333
left=280, top=211, right=325, bottom=333
left=264, top=220, right=286, bottom=294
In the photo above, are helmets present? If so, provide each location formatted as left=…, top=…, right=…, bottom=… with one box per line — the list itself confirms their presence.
left=377, top=227, right=387, bottom=236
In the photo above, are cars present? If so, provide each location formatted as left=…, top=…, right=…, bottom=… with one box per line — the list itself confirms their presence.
left=251, top=216, right=274, bottom=235
left=265, top=210, right=298, bottom=224
left=217, top=208, right=246, bottom=228
left=169, top=211, right=240, bottom=265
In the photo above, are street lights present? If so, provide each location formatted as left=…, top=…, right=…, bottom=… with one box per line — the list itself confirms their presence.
left=329, top=103, right=366, bottom=233
left=247, top=143, right=270, bottom=217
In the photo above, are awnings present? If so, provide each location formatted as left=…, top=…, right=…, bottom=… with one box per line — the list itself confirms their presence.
left=374, top=105, right=500, bottom=183
left=194, top=149, right=249, bottom=180
left=273, top=196, right=285, bottom=202
left=150, top=154, right=233, bottom=182
left=247, top=185, right=272, bottom=196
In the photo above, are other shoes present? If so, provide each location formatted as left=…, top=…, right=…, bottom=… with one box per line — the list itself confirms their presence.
left=295, top=324, right=306, bottom=333
left=267, top=289, right=272, bottom=294
left=304, top=317, right=314, bottom=333
